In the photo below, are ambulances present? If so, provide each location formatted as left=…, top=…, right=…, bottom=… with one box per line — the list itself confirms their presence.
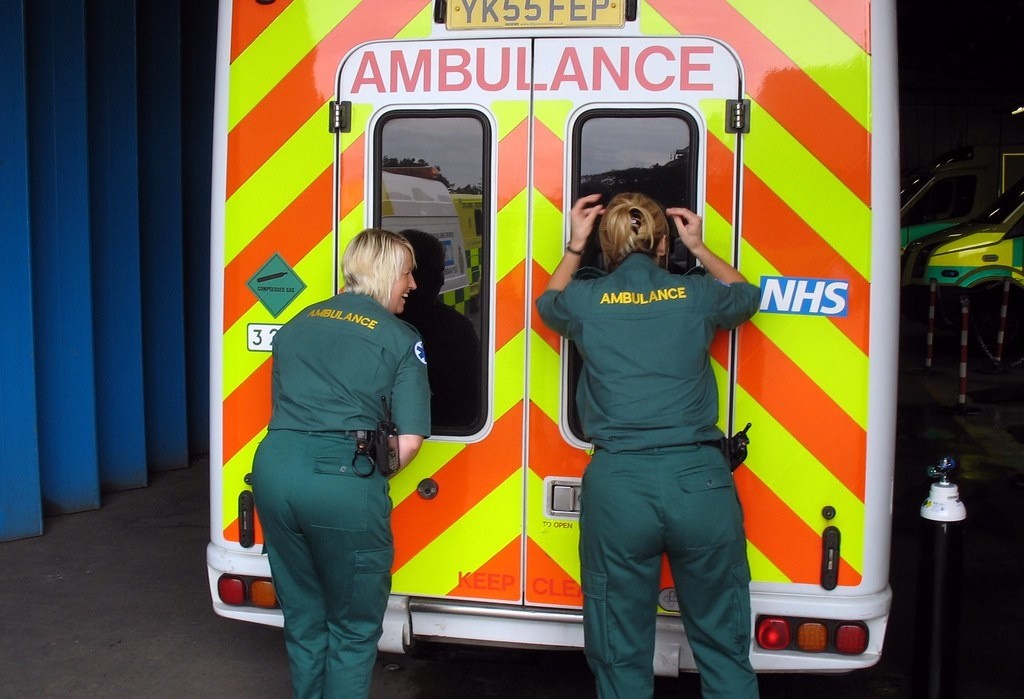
left=900, top=201, right=1024, bottom=372
left=901, top=143, right=1024, bottom=256
left=206, top=0, right=900, bottom=675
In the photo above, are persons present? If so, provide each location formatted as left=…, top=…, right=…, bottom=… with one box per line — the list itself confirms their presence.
left=399, top=230, right=479, bottom=430
left=536, top=193, right=763, bottom=699
left=251, top=229, right=431, bottom=699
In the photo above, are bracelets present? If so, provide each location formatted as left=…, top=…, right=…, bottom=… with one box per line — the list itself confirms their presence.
left=567, top=241, right=586, bottom=254
